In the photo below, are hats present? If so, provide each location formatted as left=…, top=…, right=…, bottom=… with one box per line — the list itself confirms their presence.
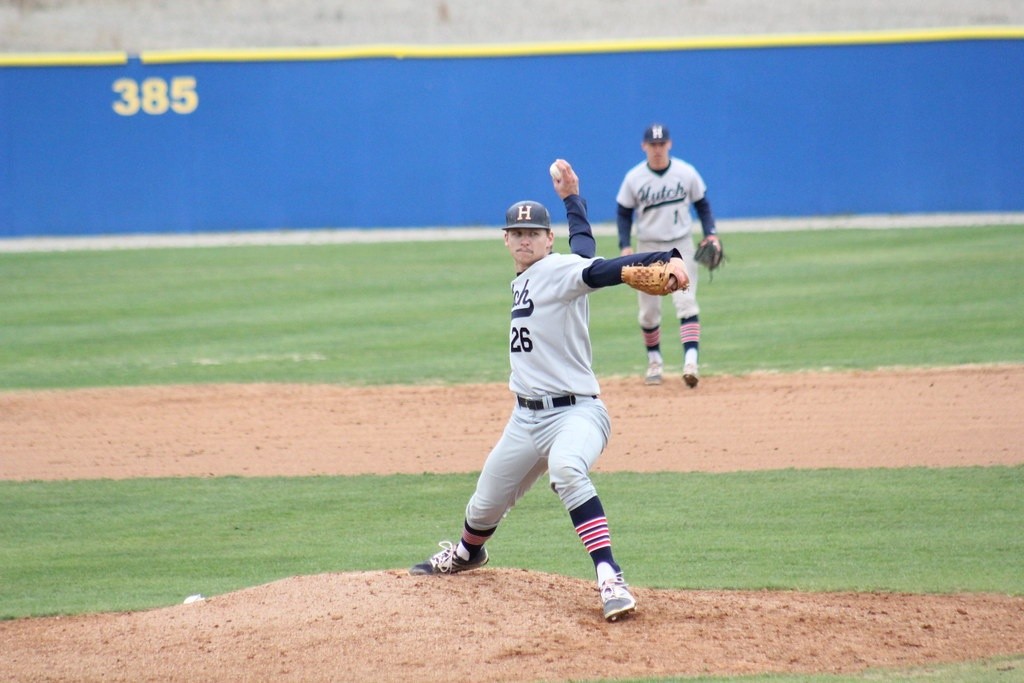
left=644, top=125, right=668, bottom=141
left=501, top=201, right=550, bottom=229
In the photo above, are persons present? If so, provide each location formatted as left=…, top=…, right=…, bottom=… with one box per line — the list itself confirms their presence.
left=410, top=158, right=693, bottom=622
left=615, top=122, right=725, bottom=389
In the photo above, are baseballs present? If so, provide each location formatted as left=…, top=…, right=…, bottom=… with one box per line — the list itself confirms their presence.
left=549, top=161, right=562, bottom=180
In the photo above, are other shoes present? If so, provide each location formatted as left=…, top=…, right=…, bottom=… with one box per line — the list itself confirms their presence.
left=683, top=369, right=698, bottom=388
left=645, top=366, right=663, bottom=385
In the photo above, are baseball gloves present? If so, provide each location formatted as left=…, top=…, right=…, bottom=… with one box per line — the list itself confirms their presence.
left=692, top=233, right=723, bottom=271
left=620, top=256, right=692, bottom=297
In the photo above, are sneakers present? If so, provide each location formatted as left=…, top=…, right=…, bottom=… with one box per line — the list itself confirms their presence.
left=409, top=541, right=489, bottom=575
left=599, top=572, right=636, bottom=621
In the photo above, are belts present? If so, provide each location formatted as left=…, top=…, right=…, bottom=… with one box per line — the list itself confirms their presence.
left=518, top=393, right=597, bottom=410
